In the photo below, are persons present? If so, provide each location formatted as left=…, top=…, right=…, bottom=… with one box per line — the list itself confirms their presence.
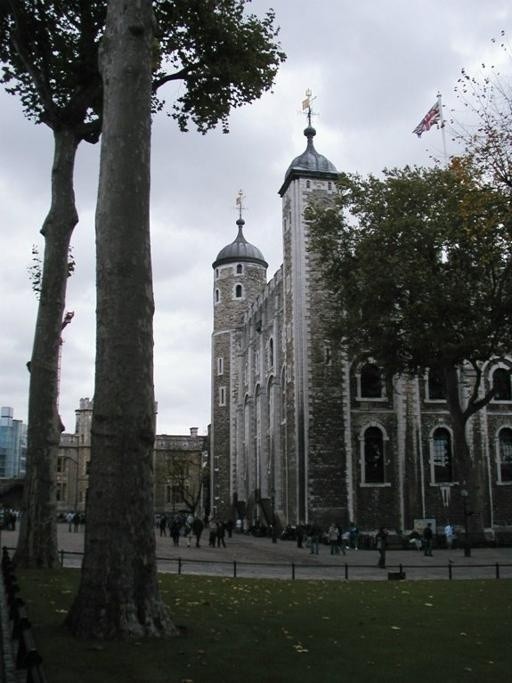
left=73, top=511, right=81, bottom=532
left=344, top=522, right=358, bottom=551
left=296, top=517, right=305, bottom=548
left=327, top=521, right=341, bottom=555
left=444, top=518, right=457, bottom=563
left=10, top=508, right=17, bottom=530
left=374, top=526, right=389, bottom=567
left=308, top=518, right=322, bottom=554
left=422, top=522, right=434, bottom=556
left=5, top=508, right=12, bottom=530
left=153, top=509, right=234, bottom=548
left=408, top=526, right=423, bottom=550
left=65, top=510, right=75, bottom=532
left=332, top=522, right=346, bottom=555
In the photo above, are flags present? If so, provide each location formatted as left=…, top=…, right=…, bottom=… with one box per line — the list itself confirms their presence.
left=411, top=100, right=441, bottom=138
